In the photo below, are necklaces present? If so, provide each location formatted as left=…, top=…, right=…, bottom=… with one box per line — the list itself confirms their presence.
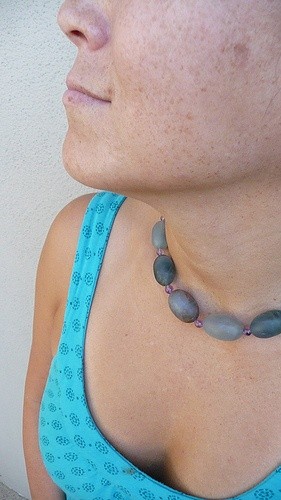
left=150, top=214, right=281, bottom=350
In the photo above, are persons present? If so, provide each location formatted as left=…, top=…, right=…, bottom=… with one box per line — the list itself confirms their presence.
left=19, top=0, right=280, bottom=497
